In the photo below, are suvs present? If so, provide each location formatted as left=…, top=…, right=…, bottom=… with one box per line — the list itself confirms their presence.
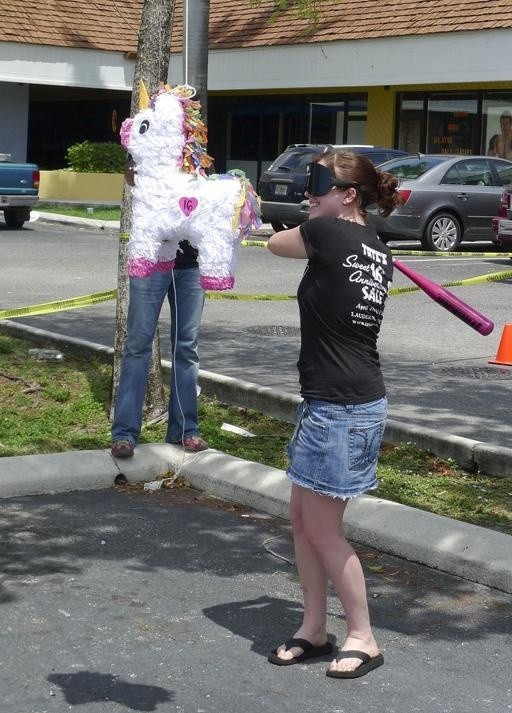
left=257, top=141, right=413, bottom=235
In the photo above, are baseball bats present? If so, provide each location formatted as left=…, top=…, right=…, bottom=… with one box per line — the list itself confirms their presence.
left=390, top=254, right=495, bottom=335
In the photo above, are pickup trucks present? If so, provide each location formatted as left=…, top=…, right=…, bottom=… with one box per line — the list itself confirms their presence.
left=1, top=150, right=41, bottom=229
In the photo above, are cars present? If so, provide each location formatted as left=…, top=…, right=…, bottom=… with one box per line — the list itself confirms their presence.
left=363, top=152, right=511, bottom=250
left=490, top=187, right=512, bottom=248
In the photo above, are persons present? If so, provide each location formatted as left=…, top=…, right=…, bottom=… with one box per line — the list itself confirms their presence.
left=111, top=150, right=208, bottom=458
left=487, top=110, right=512, bottom=162
left=267, top=148, right=400, bottom=679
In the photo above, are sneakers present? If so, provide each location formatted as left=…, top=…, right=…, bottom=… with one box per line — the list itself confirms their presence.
left=180, top=435, right=209, bottom=452
left=110, top=439, right=134, bottom=457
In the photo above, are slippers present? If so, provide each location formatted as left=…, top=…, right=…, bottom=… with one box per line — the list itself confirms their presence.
left=268, top=637, right=332, bottom=666
left=325, top=650, right=384, bottom=679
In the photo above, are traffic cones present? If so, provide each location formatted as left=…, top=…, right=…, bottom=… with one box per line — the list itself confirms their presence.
left=487, top=320, right=512, bottom=366
